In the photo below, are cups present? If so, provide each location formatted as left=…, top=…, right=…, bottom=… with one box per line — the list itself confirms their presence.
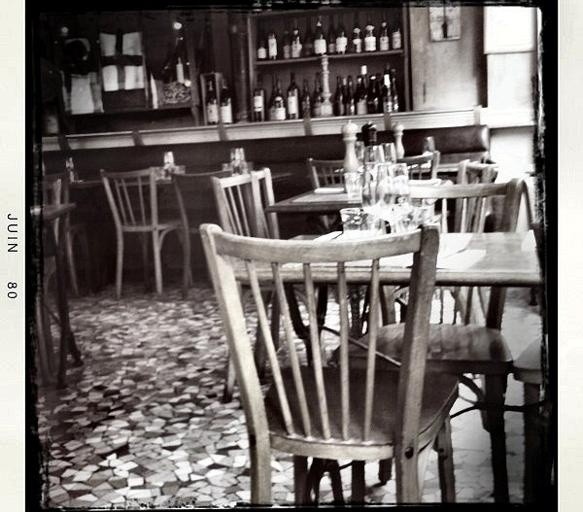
left=339, top=164, right=412, bottom=241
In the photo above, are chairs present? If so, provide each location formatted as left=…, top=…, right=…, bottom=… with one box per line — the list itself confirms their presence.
left=39, top=149, right=548, bottom=509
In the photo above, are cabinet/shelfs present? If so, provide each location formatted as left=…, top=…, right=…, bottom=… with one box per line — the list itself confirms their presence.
left=239, top=3, right=410, bottom=121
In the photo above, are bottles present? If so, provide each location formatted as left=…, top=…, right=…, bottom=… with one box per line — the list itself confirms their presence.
left=254, top=15, right=402, bottom=122
left=164, top=152, right=173, bottom=169
left=229, top=147, right=246, bottom=177
left=206, top=77, right=234, bottom=124
left=64, top=157, right=74, bottom=183
